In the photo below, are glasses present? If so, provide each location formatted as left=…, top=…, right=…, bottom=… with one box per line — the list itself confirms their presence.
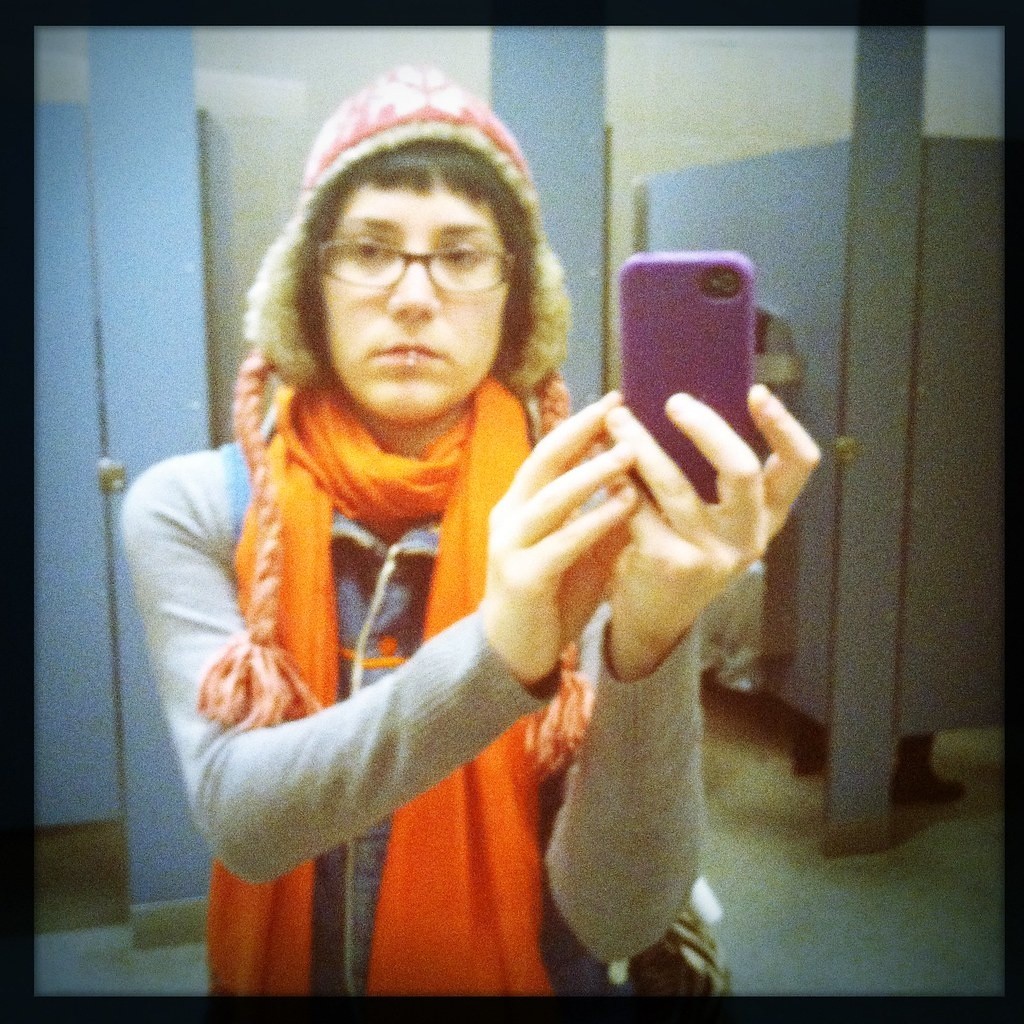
left=318, top=237, right=513, bottom=296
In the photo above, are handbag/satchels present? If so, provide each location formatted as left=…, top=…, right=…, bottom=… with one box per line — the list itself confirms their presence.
left=541, top=889, right=731, bottom=997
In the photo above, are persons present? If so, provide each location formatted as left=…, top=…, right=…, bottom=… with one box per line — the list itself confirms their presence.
left=116, top=50, right=824, bottom=998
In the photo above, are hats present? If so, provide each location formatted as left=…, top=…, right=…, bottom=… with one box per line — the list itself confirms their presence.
left=199, top=69, right=583, bottom=781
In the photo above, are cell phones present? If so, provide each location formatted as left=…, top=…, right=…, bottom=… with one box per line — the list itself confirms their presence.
left=620, top=252, right=760, bottom=514
